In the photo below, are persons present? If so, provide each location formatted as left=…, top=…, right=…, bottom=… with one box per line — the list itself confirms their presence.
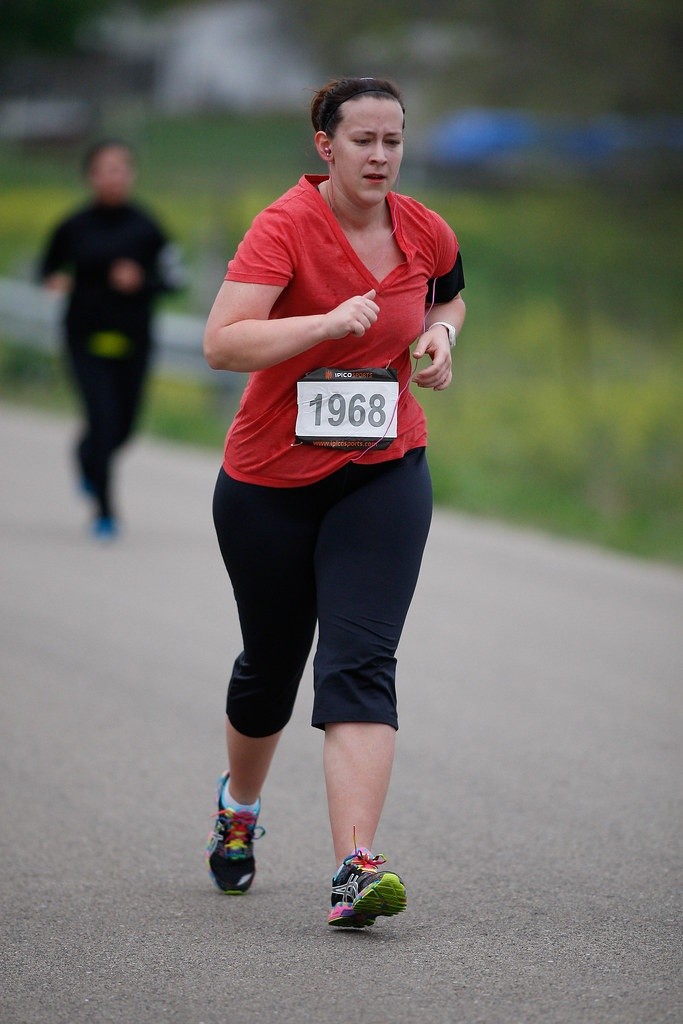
left=35, top=139, right=190, bottom=538
left=203, top=78, right=466, bottom=926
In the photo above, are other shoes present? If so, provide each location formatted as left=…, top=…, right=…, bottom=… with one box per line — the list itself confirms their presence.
left=93, top=516, right=115, bottom=548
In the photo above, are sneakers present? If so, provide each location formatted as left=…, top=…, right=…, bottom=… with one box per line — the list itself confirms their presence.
left=206, top=769, right=263, bottom=893
left=327, top=823, right=409, bottom=930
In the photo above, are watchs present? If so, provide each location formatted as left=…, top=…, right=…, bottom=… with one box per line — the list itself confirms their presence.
left=427, top=322, right=456, bottom=347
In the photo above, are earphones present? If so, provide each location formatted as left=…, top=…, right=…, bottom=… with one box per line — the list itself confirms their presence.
left=324, top=147, right=332, bottom=157
